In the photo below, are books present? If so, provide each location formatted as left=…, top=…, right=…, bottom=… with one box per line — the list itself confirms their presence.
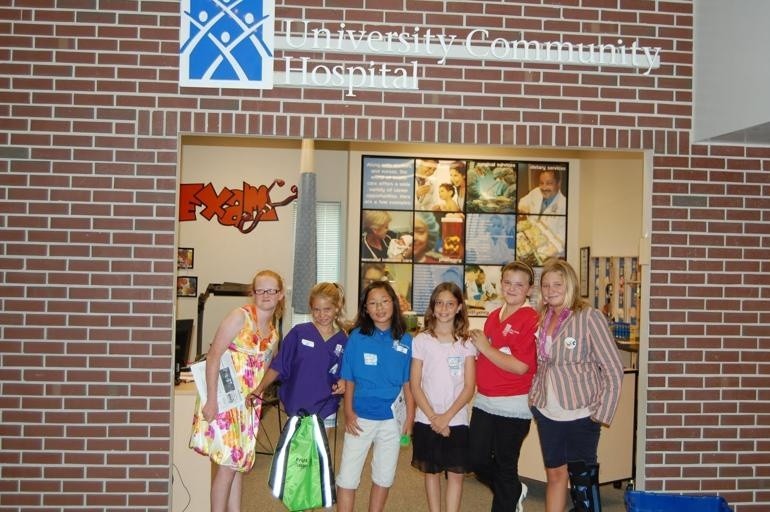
left=188, top=349, right=244, bottom=414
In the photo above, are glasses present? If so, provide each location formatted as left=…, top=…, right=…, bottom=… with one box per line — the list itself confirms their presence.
left=254, top=289, right=280, bottom=294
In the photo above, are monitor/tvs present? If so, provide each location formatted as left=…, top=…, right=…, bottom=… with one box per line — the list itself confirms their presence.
left=175, top=320, right=195, bottom=379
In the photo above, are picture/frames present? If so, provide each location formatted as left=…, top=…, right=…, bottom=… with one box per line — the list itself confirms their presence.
left=178, top=248, right=194, bottom=269
left=177, top=276, right=198, bottom=297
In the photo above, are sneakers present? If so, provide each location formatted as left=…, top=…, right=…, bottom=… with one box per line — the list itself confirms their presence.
left=516, top=482, right=529, bottom=512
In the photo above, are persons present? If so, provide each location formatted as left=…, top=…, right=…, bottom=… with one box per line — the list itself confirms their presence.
left=526, top=254, right=624, bottom=510
left=245, top=282, right=352, bottom=511
left=408, top=280, right=479, bottom=511
left=183, top=270, right=287, bottom=511
left=338, top=281, right=416, bottom=512
left=361, top=157, right=565, bottom=317
left=463, top=260, right=543, bottom=510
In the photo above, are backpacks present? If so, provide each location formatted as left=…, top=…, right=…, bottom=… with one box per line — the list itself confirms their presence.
left=268, top=409, right=336, bottom=512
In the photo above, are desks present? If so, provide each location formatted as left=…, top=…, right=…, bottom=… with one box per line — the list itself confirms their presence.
left=517, top=368, right=639, bottom=489
left=171, top=381, right=212, bottom=512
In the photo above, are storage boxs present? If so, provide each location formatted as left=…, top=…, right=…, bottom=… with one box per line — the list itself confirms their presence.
left=624, top=490, right=734, bottom=512
